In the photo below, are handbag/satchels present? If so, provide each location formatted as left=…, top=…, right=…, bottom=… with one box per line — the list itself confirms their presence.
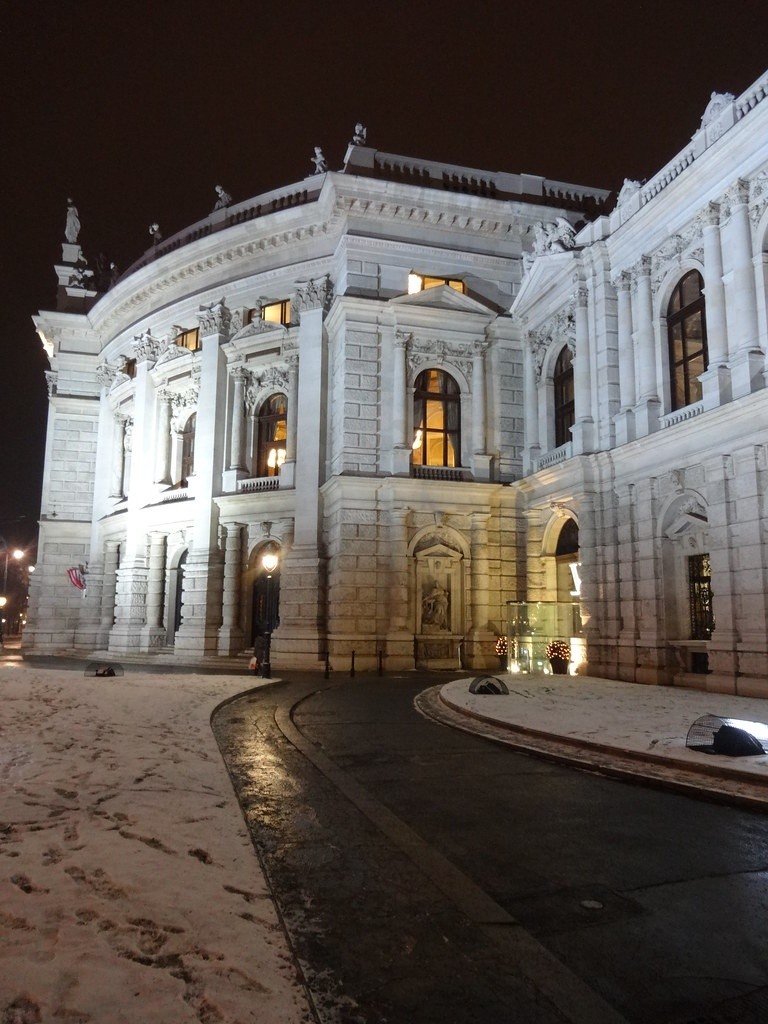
left=249, top=656, right=257, bottom=669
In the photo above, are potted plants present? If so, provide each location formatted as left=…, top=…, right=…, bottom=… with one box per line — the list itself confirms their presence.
left=495, top=636, right=515, bottom=671
left=546, top=641, right=570, bottom=674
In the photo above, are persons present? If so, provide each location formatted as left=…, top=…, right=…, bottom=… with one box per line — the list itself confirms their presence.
left=520, top=217, right=576, bottom=284
left=423, top=580, right=449, bottom=630
left=254, top=633, right=266, bottom=672
left=311, top=147, right=327, bottom=173
left=64, top=197, right=80, bottom=243
left=212, top=185, right=232, bottom=212
left=148, top=223, right=162, bottom=245
left=71, top=250, right=119, bottom=289
left=353, top=123, right=366, bottom=146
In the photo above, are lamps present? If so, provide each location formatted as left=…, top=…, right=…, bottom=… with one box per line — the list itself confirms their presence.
left=262, top=555, right=278, bottom=572
